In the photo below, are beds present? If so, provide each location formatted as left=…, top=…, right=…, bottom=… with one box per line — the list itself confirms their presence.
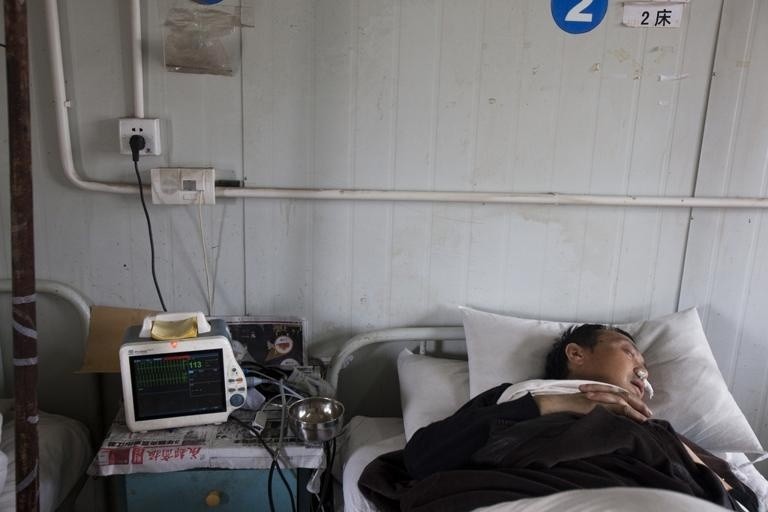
left=321, top=326, right=768, bottom=512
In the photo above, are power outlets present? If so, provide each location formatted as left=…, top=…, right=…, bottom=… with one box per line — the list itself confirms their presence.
left=118, top=118, right=162, bottom=157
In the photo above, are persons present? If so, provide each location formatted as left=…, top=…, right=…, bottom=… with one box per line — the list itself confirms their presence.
left=405, top=322, right=759, bottom=512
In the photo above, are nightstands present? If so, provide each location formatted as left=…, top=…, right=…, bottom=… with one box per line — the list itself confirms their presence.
left=95, top=403, right=325, bottom=512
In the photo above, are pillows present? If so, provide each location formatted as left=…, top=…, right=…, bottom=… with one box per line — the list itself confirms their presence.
left=456, top=303, right=765, bottom=442
left=398, top=344, right=469, bottom=448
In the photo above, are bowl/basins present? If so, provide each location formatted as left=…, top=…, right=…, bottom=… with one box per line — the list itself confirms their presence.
left=288, top=397, right=345, bottom=442
